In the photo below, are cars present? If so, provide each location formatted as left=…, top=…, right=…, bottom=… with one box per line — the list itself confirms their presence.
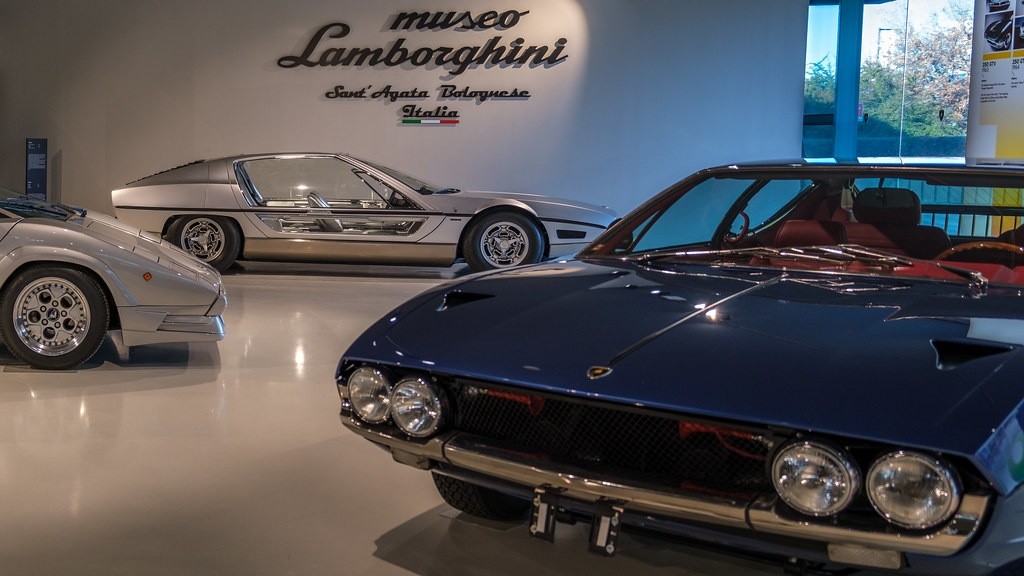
left=989, top=0, right=1011, bottom=12
left=109, top=151, right=635, bottom=275
left=334, top=156, right=1023, bottom=576
left=1, top=181, right=228, bottom=372
left=984, top=11, right=1014, bottom=51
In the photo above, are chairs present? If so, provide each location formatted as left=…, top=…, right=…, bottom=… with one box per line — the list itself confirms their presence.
left=841, top=183, right=953, bottom=262
left=307, top=191, right=363, bottom=233
left=751, top=218, right=870, bottom=275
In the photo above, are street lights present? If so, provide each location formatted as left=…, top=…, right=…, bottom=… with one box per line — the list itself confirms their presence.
left=877, top=27, right=899, bottom=61
